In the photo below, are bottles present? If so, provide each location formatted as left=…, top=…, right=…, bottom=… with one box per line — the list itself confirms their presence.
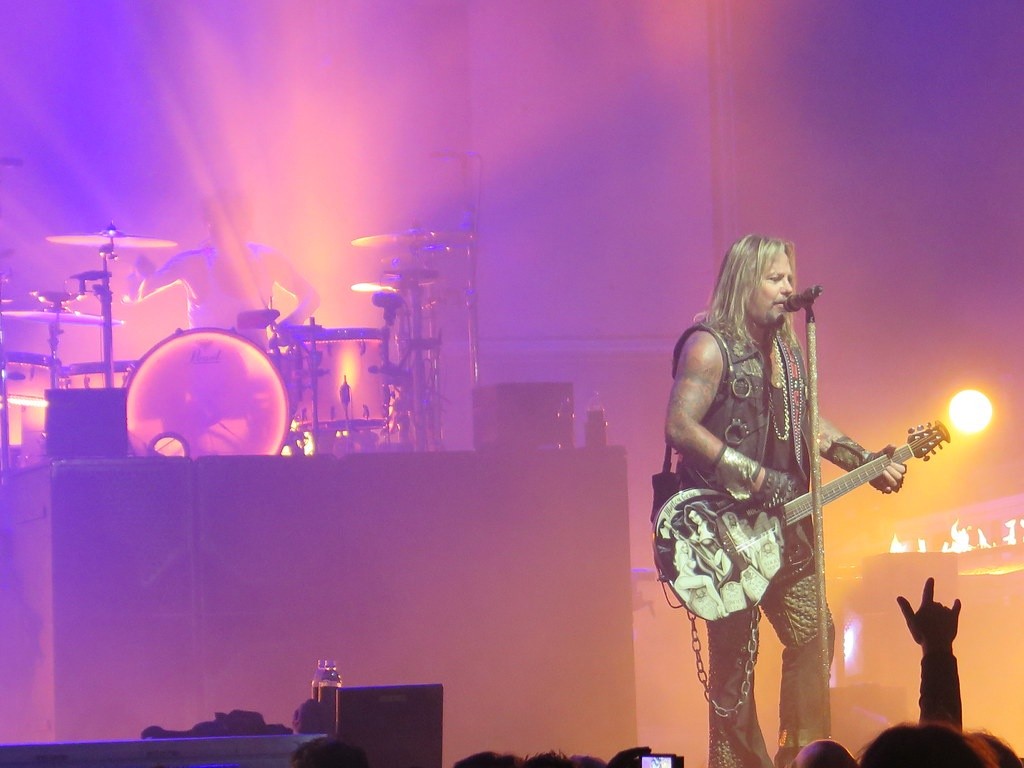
left=318, top=659, right=342, bottom=710
left=557, top=394, right=575, bottom=448
left=310, top=659, right=326, bottom=701
left=586, top=390, right=604, bottom=421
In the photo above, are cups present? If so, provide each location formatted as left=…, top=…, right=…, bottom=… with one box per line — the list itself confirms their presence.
left=584, top=422, right=608, bottom=446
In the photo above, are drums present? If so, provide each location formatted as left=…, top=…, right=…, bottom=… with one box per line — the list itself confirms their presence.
left=68, top=360, right=138, bottom=388
left=3, top=351, right=62, bottom=406
left=288, top=325, right=391, bottom=430
left=122, top=326, right=289, bottom=457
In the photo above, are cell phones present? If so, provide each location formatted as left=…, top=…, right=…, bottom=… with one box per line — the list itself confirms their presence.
left=639, top=754, right=677, bottom=768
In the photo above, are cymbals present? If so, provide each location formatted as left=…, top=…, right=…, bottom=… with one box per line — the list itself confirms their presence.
left=0, top=306, right=124, bottom=326
left=350, top=268, right=438, bottom=292
left=46, top=229, right=179, bottom=247
left=350, top=228, right=475, bottom=247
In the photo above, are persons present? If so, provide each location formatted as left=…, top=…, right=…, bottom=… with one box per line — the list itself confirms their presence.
left=665, top=234, right=905, bottom=768
left=288, top=578, right=1024, bottom=768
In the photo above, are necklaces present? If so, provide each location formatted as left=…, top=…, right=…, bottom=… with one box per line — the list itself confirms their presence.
left=767, top=335, right=790, bottom=440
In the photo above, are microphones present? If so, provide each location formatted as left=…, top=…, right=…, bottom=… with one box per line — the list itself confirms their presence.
left=70, top=271, right=112, bottom=279
left=783, top=285, right=822, bottom=312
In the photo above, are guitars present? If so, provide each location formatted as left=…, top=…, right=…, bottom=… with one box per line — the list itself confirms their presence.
left=651, top=419, right=950, bottom=624
